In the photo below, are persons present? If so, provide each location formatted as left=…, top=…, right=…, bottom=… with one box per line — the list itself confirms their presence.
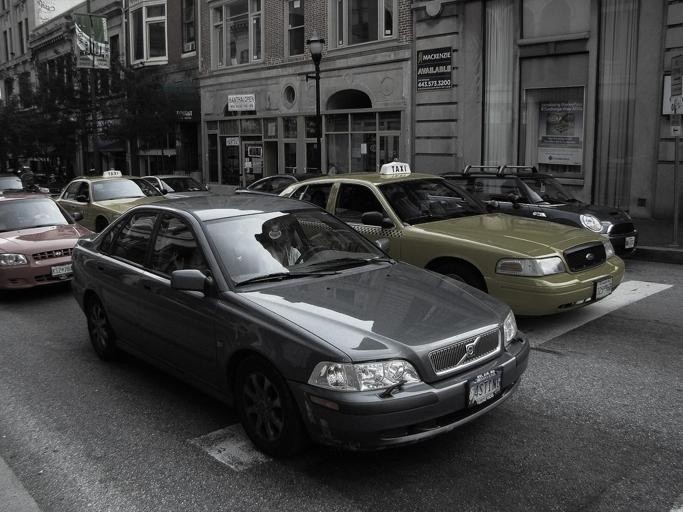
left=256, top=219, right=303, bottom=271
left=328, top=160, right=336, bottom=176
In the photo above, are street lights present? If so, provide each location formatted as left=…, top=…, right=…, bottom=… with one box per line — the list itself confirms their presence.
left=306, top=31, right=325, bottom=174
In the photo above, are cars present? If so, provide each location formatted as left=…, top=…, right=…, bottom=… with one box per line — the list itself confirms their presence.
left=55, top=170, right=180, bottom=244
left=0, top=174, right=50, bottom=193
left=0, top=188, right=97, bottom=292
left=70, top=190, right=530, bottom=461
left=427, top=164, right=639, bottom=257
left=277, top=161, right=626, bottom=319
left=141, top=175, right=210, bottom=194
left=245, top=171, right=333, bottom=208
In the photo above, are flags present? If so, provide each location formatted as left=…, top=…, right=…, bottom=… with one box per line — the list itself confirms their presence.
left=74, top=12, right=111, bottom=70
